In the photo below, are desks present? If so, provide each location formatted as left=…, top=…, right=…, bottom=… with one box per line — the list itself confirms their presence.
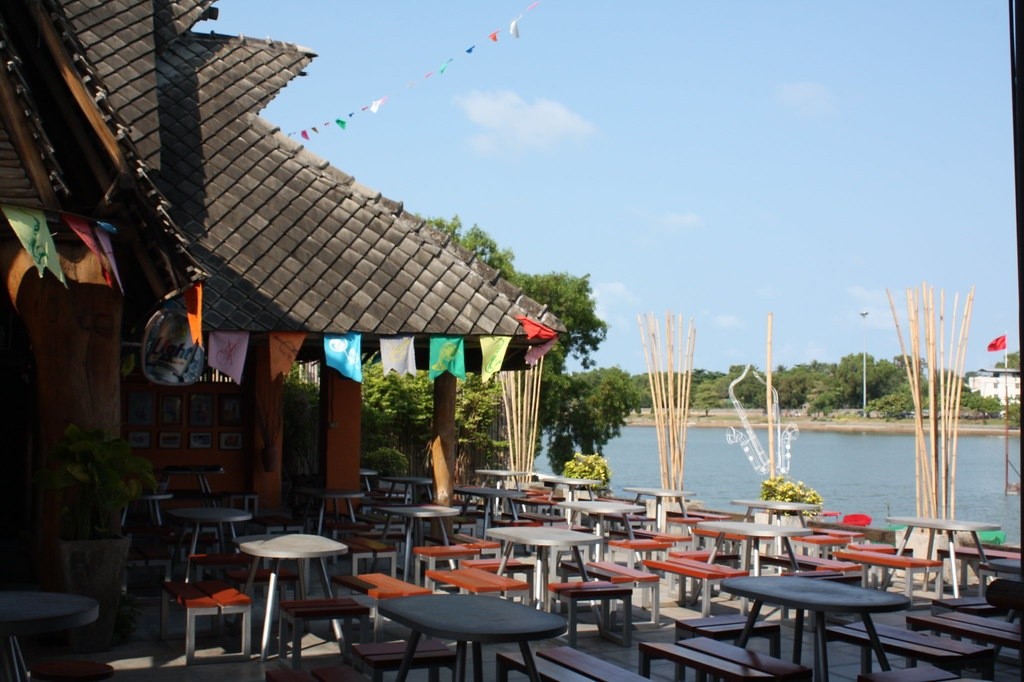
left=881, top=515, right=1001, bottom=600
left=730, top=499, right=819, bottom=557
left=690, top=521, right=815, bottom=606
left=122, top=465, right=695, bottom=682
left=719, top=576, right=912, bottom=682
left=0, top=591, right=100, bottom=682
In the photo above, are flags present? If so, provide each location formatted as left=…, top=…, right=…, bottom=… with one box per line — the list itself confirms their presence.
left=987, top=335, right=1006, bottom=352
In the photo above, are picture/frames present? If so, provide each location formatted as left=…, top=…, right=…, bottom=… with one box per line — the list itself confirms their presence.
left=126, top=391, right=243, bottom=451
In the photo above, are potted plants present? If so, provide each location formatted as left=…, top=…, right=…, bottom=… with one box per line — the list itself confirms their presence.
left=41, top=421, right=160, bottom=654
left=561, top=452, right=610, bottom=526
left=753, top=477, right=825, bottom=557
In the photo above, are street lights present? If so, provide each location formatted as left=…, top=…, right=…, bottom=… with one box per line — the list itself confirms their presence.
left=860, top=311, right=871, bottom=419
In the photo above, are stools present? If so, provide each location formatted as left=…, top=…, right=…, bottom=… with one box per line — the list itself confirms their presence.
left=30, top=660, right=114, bottom=682
left=130, top=485, right=1023, bottom=681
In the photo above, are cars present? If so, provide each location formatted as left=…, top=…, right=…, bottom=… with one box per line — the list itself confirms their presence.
left=903, top=406, right=1002, bottom=422
